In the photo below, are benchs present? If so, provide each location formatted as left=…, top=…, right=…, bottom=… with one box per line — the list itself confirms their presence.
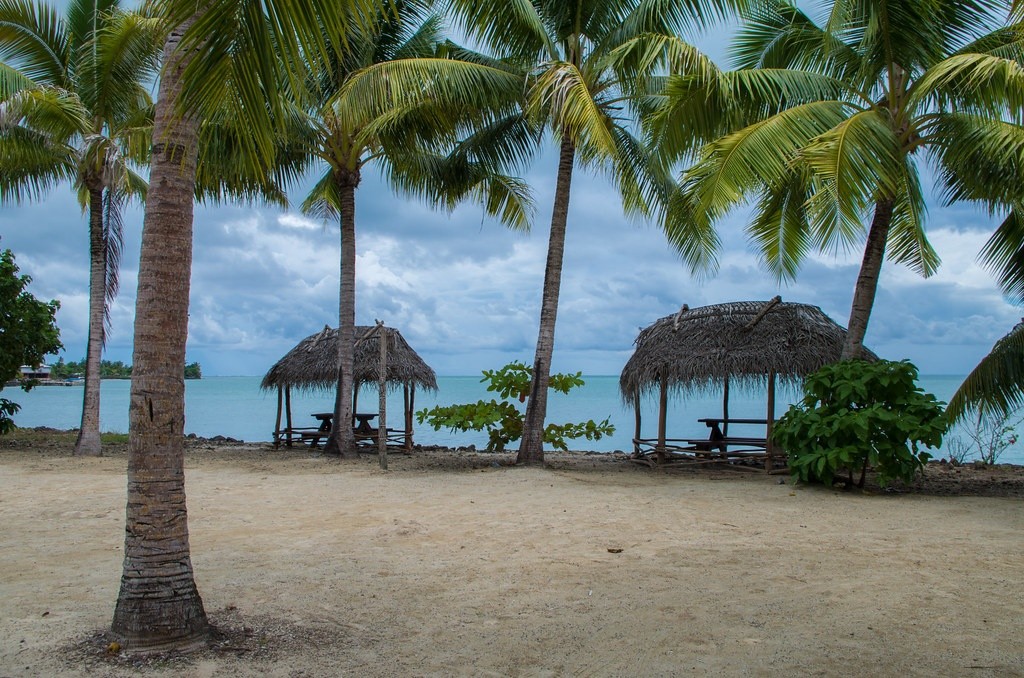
left=687, top=437, right=769, bottom=467
left=287, top=427, right=393, bottom=453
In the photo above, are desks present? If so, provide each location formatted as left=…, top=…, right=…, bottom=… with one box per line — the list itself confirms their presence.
left=308, top=413, right=380, bottom=452
left=698, top=418, right=781, bottom=460
